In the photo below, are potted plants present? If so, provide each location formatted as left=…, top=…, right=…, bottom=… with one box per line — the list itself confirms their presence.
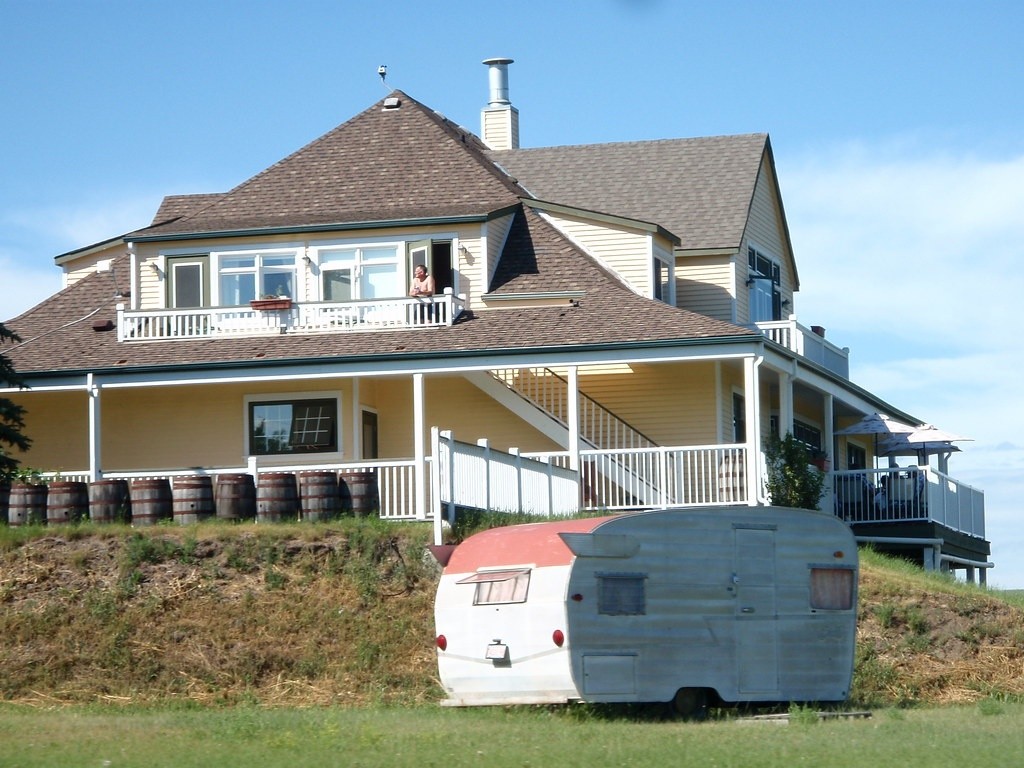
left=811, top=326, right=825, bottom=338
left=250, top=295, right=291, bottom=310
left=12, top=467, right=40, bottom=488
left=812, top=449, right=828, bottom=471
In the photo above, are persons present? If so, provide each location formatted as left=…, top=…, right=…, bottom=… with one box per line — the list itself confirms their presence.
left=874, top=463, right=925, bottom=519
left=409, top=264, right=435, bottom=320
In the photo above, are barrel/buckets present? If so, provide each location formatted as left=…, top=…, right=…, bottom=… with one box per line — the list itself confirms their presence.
left=256, top=474, right=299, bottom=522
left=173, top=476, right=214, bottom=524
left=339, top=472, right=380, bottom=520
left=300, top=472, right=337, bottom=524
left=131, top=479, right=174, bottom=525
left=719, top=453, right=748, bottom=502
left=215, top=474, right=256, bottom=521
left=89, top=480, right=133, bottom=524
left=47, top=482, right=89, bottom=524
left=9, top=483, right=48, bottom=529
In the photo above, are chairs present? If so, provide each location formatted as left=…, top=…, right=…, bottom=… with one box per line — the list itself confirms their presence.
left=836, top=474, right=928, bottom=521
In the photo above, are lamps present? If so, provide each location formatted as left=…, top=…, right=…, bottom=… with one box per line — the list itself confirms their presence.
left=782, top=300, right=790, bottom=310
left=302, top=255, right=310, bottom=266
left=745, top=277, right=756, bottom=290
left=149, top=262, right=158, bottom=272
left=458, top=244, right=467, bottom=253
left=95, top=259, right=124, bottom=297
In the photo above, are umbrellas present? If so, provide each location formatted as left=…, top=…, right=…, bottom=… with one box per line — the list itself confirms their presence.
left=833, top=412, right=915, bottom=488
left=874, top=424, right=975, bottom=467
left=872, top=442, right=963, bottom=465
